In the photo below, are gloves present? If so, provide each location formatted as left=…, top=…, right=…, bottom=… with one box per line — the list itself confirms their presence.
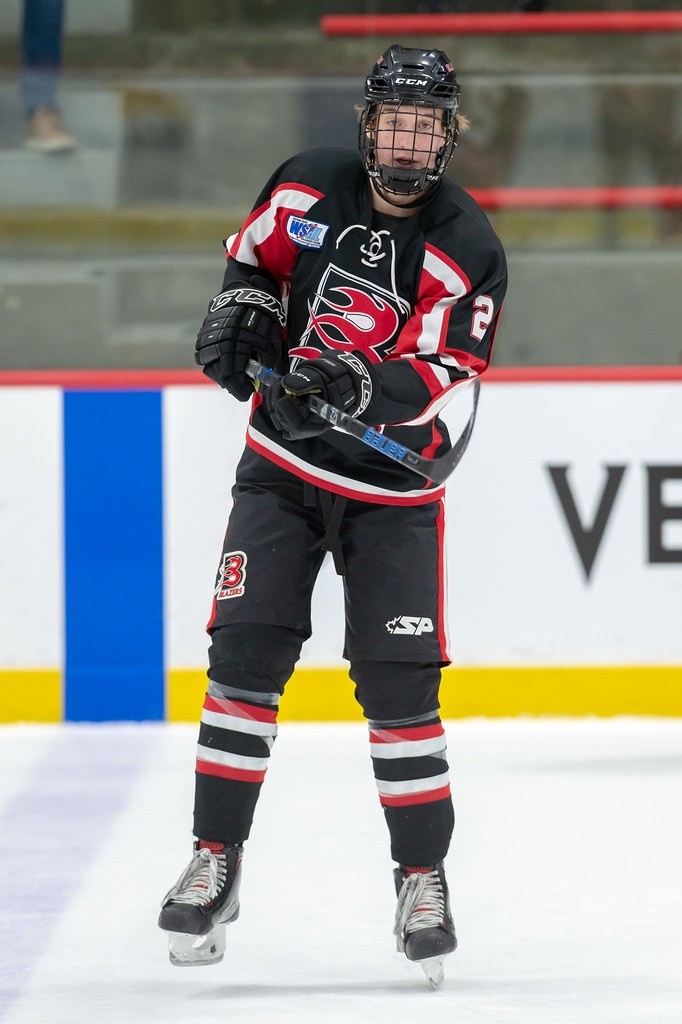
left=260, top=348, right=380, bottom=442
left=194, top=281, right=284, bottom=402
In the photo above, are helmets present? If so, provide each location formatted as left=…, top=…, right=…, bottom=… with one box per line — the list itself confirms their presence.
left=358, top=43, right=463, bottom=197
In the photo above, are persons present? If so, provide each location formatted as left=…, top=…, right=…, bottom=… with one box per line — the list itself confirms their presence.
left=159, top=43, right=507, bottom=989
left=21, top=1, right=75, bottom=154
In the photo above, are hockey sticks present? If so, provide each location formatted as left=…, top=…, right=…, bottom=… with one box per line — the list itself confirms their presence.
left=244, top=359, right=482, bottom=488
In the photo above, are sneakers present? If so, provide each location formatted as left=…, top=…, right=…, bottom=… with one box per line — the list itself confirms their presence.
left=157, top=838, right=244, bottom=969
left=392, top=863, right=458, bottom=989
left=23, top=104, right=77, bottom=153
